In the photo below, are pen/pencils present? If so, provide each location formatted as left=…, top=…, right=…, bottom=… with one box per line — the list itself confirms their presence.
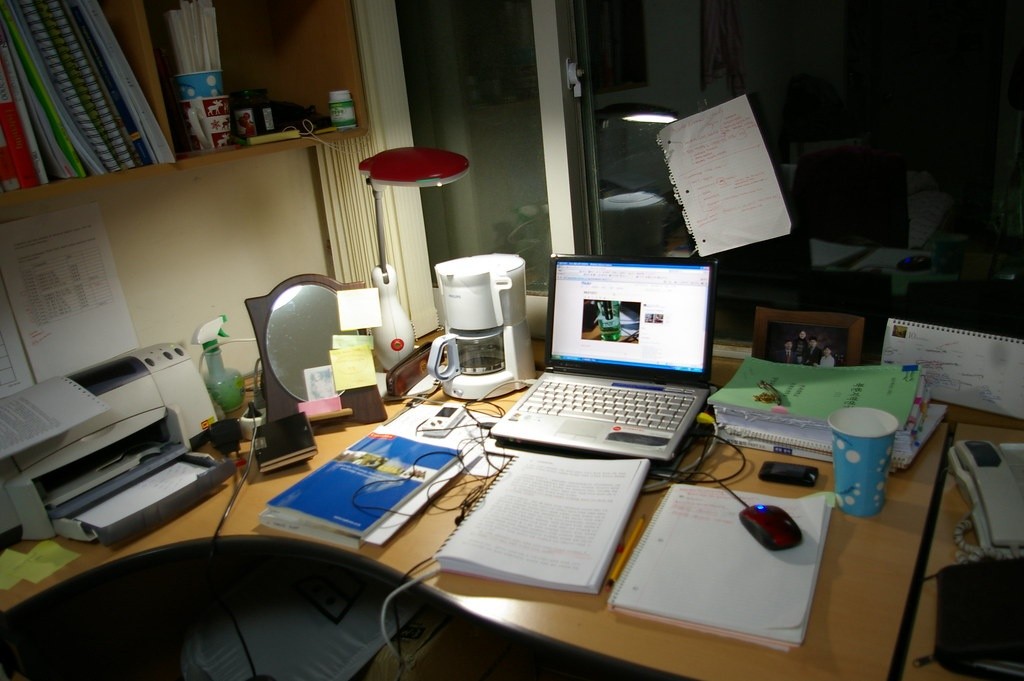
left=605, top=513, right=648, bottom=593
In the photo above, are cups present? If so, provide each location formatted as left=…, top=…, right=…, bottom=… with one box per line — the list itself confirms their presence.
left=174, top=70, right=224, bottom=100
left=827, top=408, right=899, bottom=517
left=179, top=95, right=233, bottom=151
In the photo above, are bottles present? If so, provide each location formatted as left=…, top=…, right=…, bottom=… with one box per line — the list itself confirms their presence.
left=597, top=301, right=621, bottom=341
left=327, top=90, right=356, bottom=128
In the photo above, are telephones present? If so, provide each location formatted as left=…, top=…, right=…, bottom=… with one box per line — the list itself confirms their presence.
left=946, top=437, right=1024, bottom=550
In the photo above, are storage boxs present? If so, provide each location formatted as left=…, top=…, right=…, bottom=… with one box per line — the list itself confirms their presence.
left=354, top=603, right=540, bottom=681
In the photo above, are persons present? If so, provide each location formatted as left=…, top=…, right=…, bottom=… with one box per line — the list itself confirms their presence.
left=775, top=330, right=834, bottom=366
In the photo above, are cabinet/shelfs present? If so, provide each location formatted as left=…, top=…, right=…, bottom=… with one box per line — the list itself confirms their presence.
left=419, top=0, right=650, bottom=114
left=0, top=0, right=370, bottom=208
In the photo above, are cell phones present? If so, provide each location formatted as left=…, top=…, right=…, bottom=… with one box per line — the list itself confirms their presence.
left=422, top=402, right=465, bottom=438
left=758, top=461, right=819, bottom=487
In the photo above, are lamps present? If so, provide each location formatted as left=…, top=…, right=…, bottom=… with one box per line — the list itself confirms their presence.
left=358, top=145, right=470, bottom=372
left=593, top=101, right=679, bottom=130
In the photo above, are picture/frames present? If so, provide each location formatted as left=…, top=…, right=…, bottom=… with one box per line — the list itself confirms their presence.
left=750, top=307, right=864, bottom=367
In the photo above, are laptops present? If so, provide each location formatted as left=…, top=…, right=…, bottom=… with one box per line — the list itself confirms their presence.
left=490, top=254, right=719, bottom=467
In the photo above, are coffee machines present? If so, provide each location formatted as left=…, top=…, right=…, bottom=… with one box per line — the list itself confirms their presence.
left=428, top=253, right=536, bottom=399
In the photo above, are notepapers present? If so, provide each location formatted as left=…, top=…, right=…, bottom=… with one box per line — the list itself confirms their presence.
left=246, top=128, right=301, bottom=147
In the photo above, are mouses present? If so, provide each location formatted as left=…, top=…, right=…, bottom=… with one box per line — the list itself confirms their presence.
left=739, top=504, right=802, bottom=550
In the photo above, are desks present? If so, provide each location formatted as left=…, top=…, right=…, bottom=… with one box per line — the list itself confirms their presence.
left=900, top=422, right=1024, bottom=681
left=0, top=328, right=951, bottom=681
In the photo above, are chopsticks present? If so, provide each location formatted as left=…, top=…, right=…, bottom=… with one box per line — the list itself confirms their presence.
left=167, top=0, right=221, bottom=74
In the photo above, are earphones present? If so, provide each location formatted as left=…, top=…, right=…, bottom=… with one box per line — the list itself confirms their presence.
left=455, top=505, right=467, bottom=526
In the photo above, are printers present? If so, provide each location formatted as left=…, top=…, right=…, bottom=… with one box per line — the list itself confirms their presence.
left=0, top=344, right=236, bottom=548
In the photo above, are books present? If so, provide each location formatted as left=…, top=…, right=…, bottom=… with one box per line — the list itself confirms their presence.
left=708, top=354, right=950, bottom=476
left=252, top=401, right=832, bottom=652
left=0, top=0, right=176, bottom=185
